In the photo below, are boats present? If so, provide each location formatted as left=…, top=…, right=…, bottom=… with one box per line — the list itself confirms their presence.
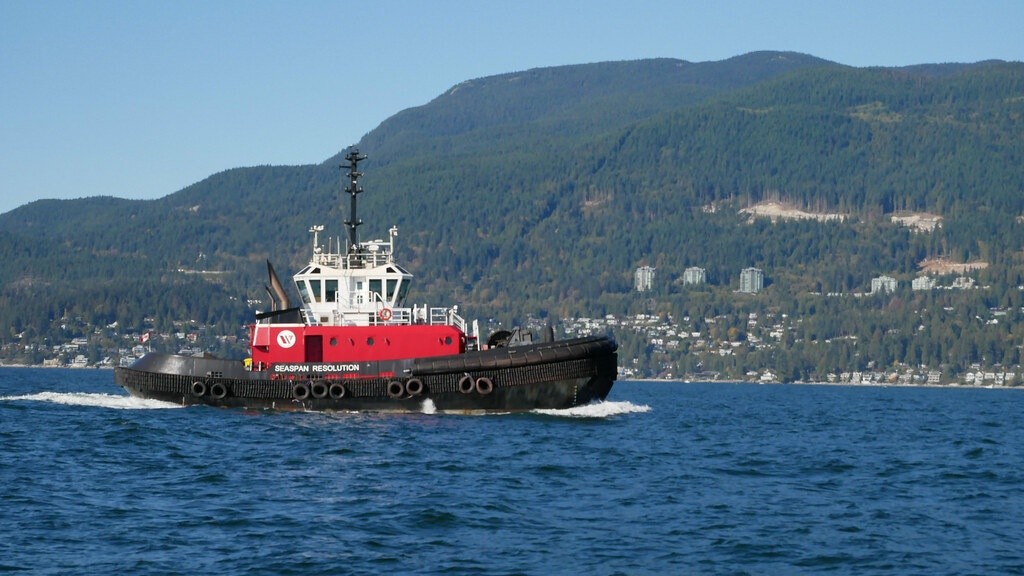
left=114, top=147, right=618, bottom=415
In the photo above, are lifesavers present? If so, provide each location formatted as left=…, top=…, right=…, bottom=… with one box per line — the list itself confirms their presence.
left=386, top=381, right=404, bottom=397
left=476, top=378, right=494, bottom=393
left=292, top=384, right=310, bottom=402
left=405, top=378, right=423, bottom=396
left=378, top=309, right=391, bottom=320
left=458, top=375, right=476, bottom=394
left=209, top=383, right=227, bottom=399
left=311, top=382, right=328, bottom=400
left=329, top=383, right=345, bottom=400
left=191, top=381, right=207, bottom=397
